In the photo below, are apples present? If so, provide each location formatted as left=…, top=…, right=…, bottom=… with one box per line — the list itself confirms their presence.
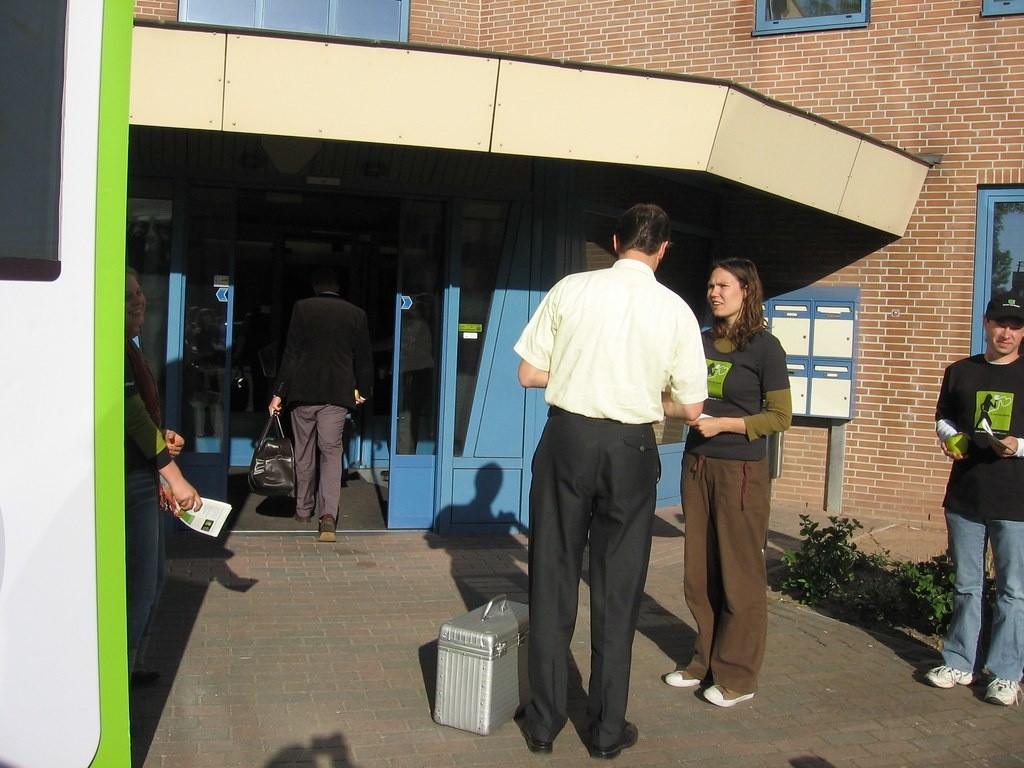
left=946, top=434, right=968, bottom=454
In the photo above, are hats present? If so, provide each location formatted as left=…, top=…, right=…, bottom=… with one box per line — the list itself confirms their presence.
left=985, top=291, right=1024, bottom=320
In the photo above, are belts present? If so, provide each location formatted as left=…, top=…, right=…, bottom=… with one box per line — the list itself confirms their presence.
left=549, top=406, right=573, bottom=415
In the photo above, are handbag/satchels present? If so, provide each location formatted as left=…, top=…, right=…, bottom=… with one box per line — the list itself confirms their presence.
left=248, top=411, right=295, bottom=496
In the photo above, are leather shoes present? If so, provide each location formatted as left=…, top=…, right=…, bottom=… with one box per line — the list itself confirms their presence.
left=589, top=721, right=639, bottom=759
left=521, top=718, right=553, bottom=753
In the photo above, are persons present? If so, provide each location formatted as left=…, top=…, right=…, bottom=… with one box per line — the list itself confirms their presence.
left=924, top=293, right=1024, bottom=706
left=125, top=266, right=203, bottom=689
left=511, top=204, right=708, bottom=760
left=231, top=312, right=254, bottom=411
left=381, top=286, right=435, bottom=482
left=182, top=303, right=224, bottom=437
left=664, top=258, right=793, bottom=708
left=269, top=263, right=374, bottom=541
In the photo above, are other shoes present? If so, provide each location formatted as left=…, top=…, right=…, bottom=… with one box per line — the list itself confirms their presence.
left=318, top=514, right=337, bottom=542
left=131, top=670, right=162, bottom=687
left=293, top=513, right=311, bottom=523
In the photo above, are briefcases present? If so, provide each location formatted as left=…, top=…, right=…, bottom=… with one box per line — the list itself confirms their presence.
left=433, top=594, right=529, bottom=735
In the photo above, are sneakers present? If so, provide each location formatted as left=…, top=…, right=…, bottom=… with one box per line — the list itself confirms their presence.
left=924, top=664, right=977, bottom=688
left=665, top=670, right=702, bottom=687
left=984, top=675, right=1024, bottom=706
left=703, top=684, right=755, bottom=708
